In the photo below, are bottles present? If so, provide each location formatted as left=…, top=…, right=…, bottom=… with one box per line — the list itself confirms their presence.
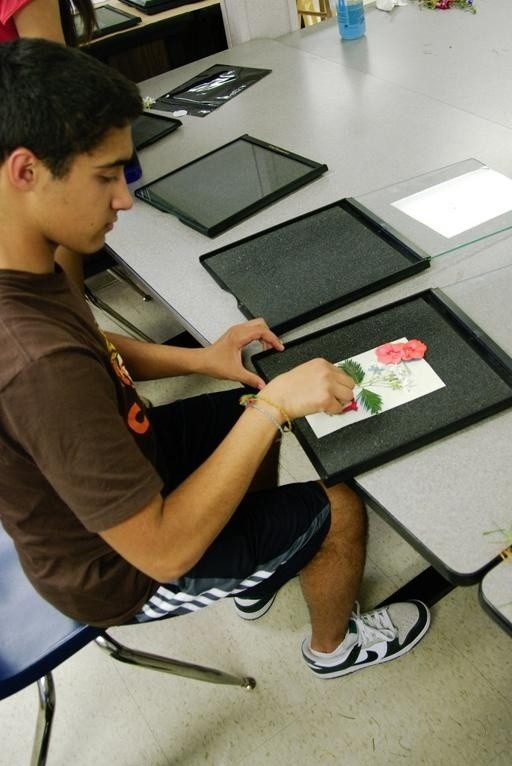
left=118, top=138, right=144, bottom=184
left=337, top=1, right=368, bottom=43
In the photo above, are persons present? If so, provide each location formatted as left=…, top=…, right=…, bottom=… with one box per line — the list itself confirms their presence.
left=0, top=0, right=100, bottom=48
left=1, top=37, right=432, bottom=678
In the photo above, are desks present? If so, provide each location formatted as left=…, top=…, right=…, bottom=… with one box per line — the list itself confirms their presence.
left=106, top=1, right=509, bottom=587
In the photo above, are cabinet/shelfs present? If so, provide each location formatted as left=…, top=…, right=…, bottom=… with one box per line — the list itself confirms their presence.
left=64, top=0, right=231, bottom=85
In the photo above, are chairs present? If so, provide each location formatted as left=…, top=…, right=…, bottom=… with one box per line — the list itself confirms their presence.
left=0, top=544, right=259, bottom=766
left=67, top=245, right=155, bottom=344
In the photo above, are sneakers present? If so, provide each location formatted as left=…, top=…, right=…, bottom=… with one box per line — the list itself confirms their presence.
left=301, top=598, right=431, bottom=680
left=234, top=592, right=277, bottom=621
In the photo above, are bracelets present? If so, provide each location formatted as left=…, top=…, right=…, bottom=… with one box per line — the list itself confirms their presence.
left=241, top=393, right=291, bottom=433
left=240, top=401, right=286, bottom=441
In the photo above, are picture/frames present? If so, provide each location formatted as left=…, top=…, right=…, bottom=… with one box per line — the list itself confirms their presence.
left=200, top=198, right=432, bottom=335
left=133, top=132, right=326, bottom=240
left=129, top=111, right=179, bottom=149
left=250, top=290, right=512, bottom=486
